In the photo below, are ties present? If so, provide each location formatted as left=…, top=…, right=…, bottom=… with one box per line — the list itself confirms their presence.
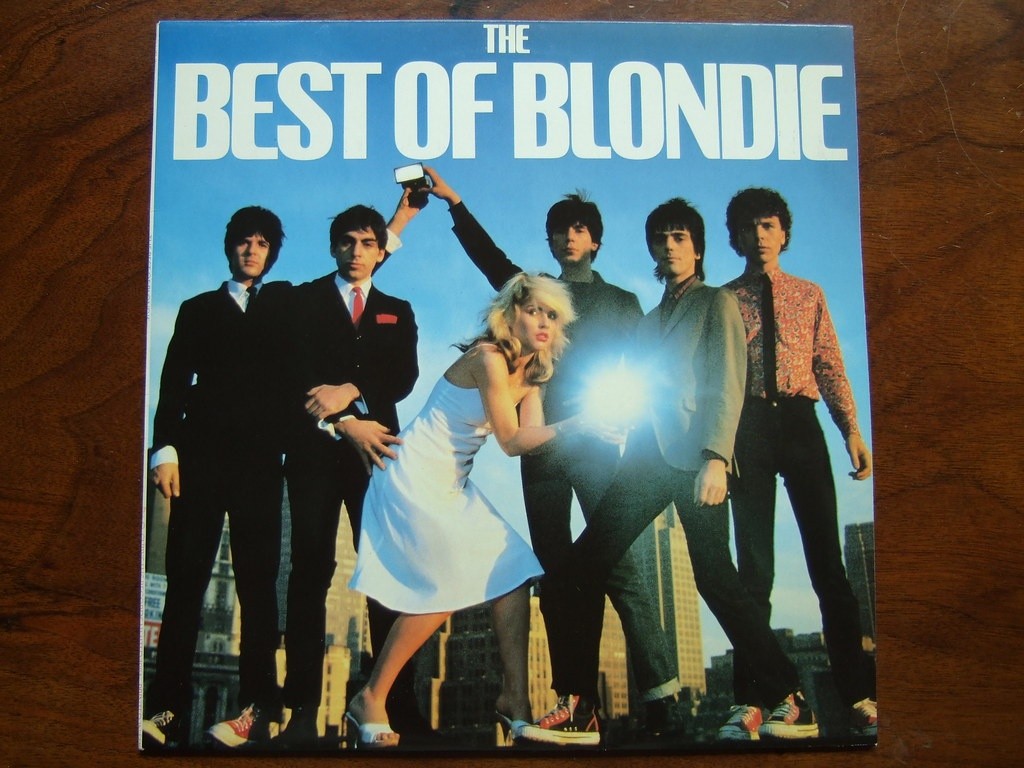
left=658, top=293, right=675, bottom=338
left=760, top=273, right=778, bottom=400
left=245, top=286, right=257, bottom=314
left=352, top=287, right=363, bottom=335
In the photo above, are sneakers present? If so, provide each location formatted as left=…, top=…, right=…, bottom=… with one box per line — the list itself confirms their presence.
left=717, top=705, right=764, bottom=740
left=849, top=698, right=877, bottom=736
left=208, top=704, right=279, bottom=748
left=758, top=689, right=819, bottom=739
left=514, top=694, right=600, bottom=745
left=143, top=711, right=181, bottom=749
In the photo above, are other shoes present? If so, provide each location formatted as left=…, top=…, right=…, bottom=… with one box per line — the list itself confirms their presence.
left=385, top=691, right=443, bottom=737
left=270, top=707, right=318, bottom=748
left=637, top=688, right=692, bottom=743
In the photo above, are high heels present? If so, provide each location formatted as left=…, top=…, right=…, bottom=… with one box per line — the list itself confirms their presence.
left=346, top=712, right=398, bottom=749
left=495, top=710, right=534, bottom=747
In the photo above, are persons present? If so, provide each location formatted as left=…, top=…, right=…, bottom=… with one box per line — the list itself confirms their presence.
left=416, top=165, right=690, bottom=746
left=717, top=188, right=878, bottom=742
left=340, top=271, right=628, bottom=750
left=267, top=204, right=418, bottom=751
left=511, top=196, right=819, bottom=747
left=141, top=187, right=422, bottom=750
